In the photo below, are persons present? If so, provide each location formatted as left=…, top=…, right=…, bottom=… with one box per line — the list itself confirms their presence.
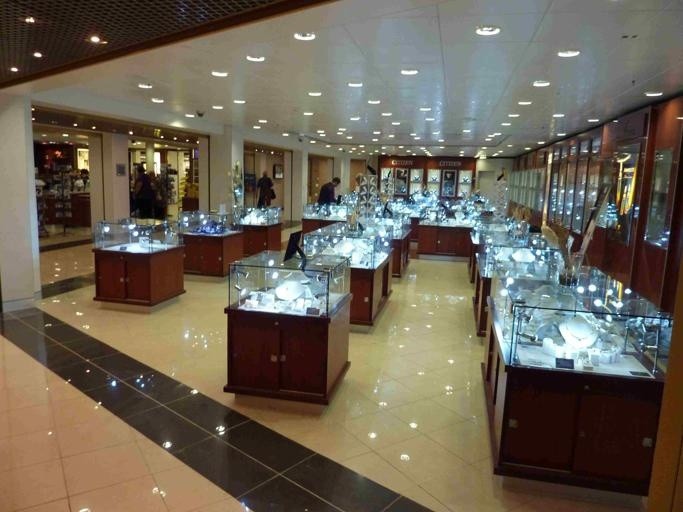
left=255, top=171, right=274, bottom=208
left=529, top=224, right=559, bottom=246
left=134, top=166, right=154, bottom=217
left=355, top=173, right=365, bottom=193
left=318, top=177, right=340, bottom=204
left=72, top=169, right=89, bottom=194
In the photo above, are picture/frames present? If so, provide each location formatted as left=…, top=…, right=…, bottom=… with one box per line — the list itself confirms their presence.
left=272, top=165, right=284, bottom=180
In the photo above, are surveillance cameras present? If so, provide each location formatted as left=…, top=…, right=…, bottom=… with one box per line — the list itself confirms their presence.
left=196, top=110, right=206, bottom=117
left=298, top=134, right=304, bottom=142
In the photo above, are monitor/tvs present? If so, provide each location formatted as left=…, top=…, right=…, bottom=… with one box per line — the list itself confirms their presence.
left=274, top=164, right=283, bottom=179
left=192, top=149, right=198, bottom=160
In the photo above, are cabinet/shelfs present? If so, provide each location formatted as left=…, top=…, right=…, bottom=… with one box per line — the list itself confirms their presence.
left=377, top=155, right=476, bottom=204
left=510, top=96, right=683, bottom=310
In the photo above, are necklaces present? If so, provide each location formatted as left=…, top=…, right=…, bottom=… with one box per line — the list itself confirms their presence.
left=565, top=318, right=596, bottom=343
left=286, top=287, right=289, bottom=291
